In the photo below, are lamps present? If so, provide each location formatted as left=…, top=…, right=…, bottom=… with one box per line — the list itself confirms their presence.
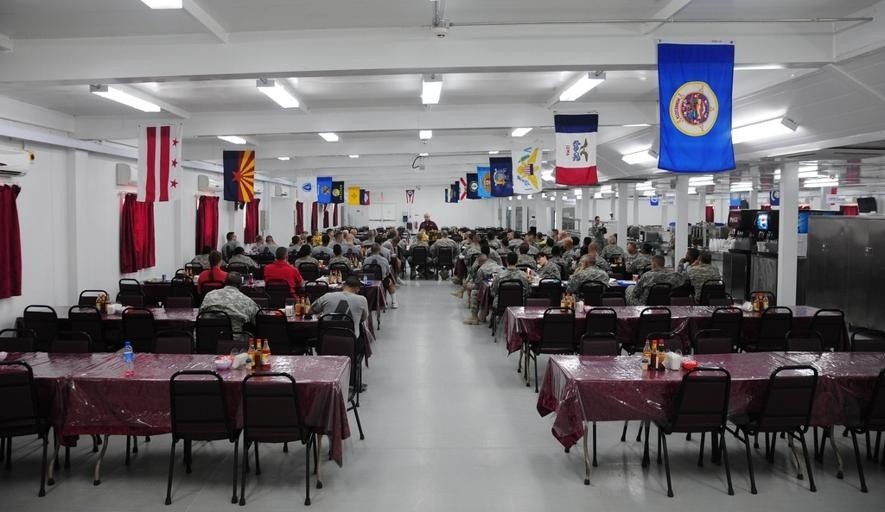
left=88, top=78, right=161, bottom=117
left=253, top=77, right=300, bottom=110
left=419, top=73, right=443, bottom=110
left=555, top=71, right=606, bottom=103
left=732, top=115, right=800, bottom=145
left=619, top=149, right=658, bottom=165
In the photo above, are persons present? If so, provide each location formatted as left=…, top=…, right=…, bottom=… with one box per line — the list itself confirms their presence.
left=407, top=227, right=722, bottom=310
left=186, top=227, right=408, bottom=310
left=197, top=270, right=261, bottom=340
left=308, top=275, right=371, bottom=394
left=589, top=215, right=608, bottom=239
left=529, top=215, right=538, bottom=234
left=417, top=212, right=438, bottom=232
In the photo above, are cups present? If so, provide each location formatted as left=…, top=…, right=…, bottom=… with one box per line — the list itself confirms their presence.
left=107, top=304, right=116, bottom=315
left=708, top=238, right=727, bottom=251
left=632, top=274, right=639, bottom=281
left=576, top=301, right=584, bottom=313
left=318, top=260, right=323, bottom=268
left=285, top=305, right=293, bottom=317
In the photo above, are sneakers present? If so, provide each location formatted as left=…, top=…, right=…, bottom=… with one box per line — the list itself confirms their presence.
left=392, top=300, right=399, bottom=309
left=450, top=290, right=463, bottom=298
left=463, top=316, right=478, bottom=324
left=478, top=311, right=486, bottom=322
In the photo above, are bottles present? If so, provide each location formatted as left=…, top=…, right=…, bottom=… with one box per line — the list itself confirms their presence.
left=643, top=339, right=652, bottom=366
left=354, top=256, right=358, bottom=268
left=329, top=268, right=333, bottom=284
left=651, top=340, right=658, bottom=369
left=565, top=291, right=571, bottom=309
left=249, top=273, right=254, bottom=284
left=763, top=295, right=769, bottom=311
left=262, top=336, right=271, bottom=372
left=658, top=339, right=666, bottom=371
left=247, top=337, right=255, bottom=368
left=759, top=296, right=763, bottom=311
left=571, top=293, right=576, bottom=310
left=95, top=293, right=102, bottom=311
left=560, top=293, right=566, bottom=312
left=245, top=359, right=253, bottom=376
left=305, top=296, right=310, bottom=315
left=337, top=269, right=343, bottom=284
left=301, top=297, right=305, bottom=315
left=753, top=299, right=758, bottom=312
left=254, top=339, right=262, bottom=371
left=642, top=359, right=648, bottom=371
left=363, top=273, right=367, bottom=284
left=295, top=295, right=301, bottom=316
left=123, top=341, right=135, bottom=371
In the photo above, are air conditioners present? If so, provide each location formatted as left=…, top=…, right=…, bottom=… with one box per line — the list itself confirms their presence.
left=114, top=163, right=138, bottom=187
left=197, top=174, right=224, bottom=194
left=0, top=147, right=31, bottom=177
left=252, top=182, right=263, bottom=194
left=274, top=183, right=288, bottom=197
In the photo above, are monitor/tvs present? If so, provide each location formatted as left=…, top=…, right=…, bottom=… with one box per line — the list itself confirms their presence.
left=757, top=210, right=778, bottom=231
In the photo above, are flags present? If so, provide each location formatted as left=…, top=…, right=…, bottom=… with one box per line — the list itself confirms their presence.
left=770, top=189, right=780, bottom=205
left=650, top=197, right=659, bottom=205
left=657, top=42, right=740, bottom=177
left=295, top=175, right=373, bottom=209
left=220, top=148, right=257, bottom=204
left=488, top=155, right=513, bottom=196
left=554, top=112, right=600, bottom=186
left=134, top=120, right=185, bottom=201
left=443, top=165, right=492, bottom=203
left=509, top=144, right=546, bottom=195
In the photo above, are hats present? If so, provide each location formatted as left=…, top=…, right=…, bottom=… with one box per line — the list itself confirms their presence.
left=267, top=236, right=273, bottom=241
left=256, top=235, right=262, bottom=241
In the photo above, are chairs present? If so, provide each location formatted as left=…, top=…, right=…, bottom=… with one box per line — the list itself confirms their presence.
left=435, top=245, right=454, bottom=281
left=412, top=246, right=428, bottom=280
left=354, top=225, right=510, bottom=244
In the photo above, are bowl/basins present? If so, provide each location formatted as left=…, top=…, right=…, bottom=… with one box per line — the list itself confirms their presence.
left=214, top=359, right=232, bottom=371
